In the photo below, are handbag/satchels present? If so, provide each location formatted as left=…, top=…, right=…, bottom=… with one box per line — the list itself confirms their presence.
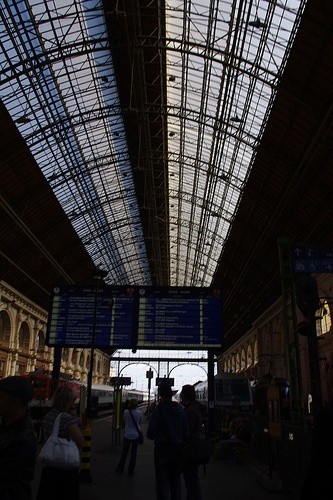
left=138, top=432, right=144, bottom=445
left=39, top=412, right=79, bottom=464
left=154, top=430, right=179, bottom=467
left=196, top=439, right=211, bottom=465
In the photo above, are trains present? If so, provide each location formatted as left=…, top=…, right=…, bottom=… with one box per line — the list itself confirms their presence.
left=14, top=372, right=143, bottom=425
left=192, top=372, right=253, bottom=415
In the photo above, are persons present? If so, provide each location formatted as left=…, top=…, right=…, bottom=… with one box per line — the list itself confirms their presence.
left=121, top=400, right=129, bottom=415
left=115, top=399, right=143, bottom=476
left=144, top=384, right=210, bottom=500
left=0, top=375, right=84, bottom=500
